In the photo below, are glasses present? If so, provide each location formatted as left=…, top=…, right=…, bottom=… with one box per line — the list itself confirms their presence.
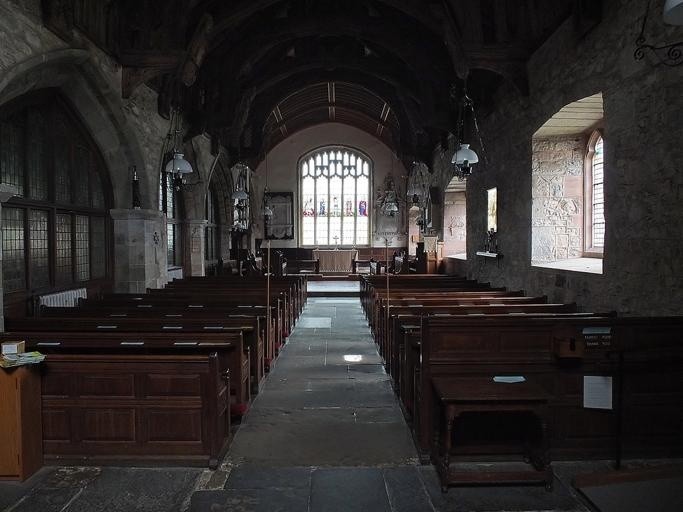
left=257, top=144, right=272, bottom=219
left=158, top=82, right=190, bottom=186
left=228, top=128, right=249, bottom=203
left=383, top=173, right=400, bottom=215
left=414, top=216, right=426, bottom=227
left=405, top=150, right=425, bottom=205
left=450, top=80, right=481, bottom=177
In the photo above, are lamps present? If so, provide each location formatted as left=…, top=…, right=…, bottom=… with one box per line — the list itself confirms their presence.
left=158, top=82, right=190, bottom=186
left=450, top=80, right=481, bottom=177
left=257, top=144, right=272, bottom=219
left=383, top=173, right=400, bottom=215
left=228, top=128, right=249, bottom=203
left=405, top=150, right=425, bottom=205
left=414, top=216, right=426, bottom=227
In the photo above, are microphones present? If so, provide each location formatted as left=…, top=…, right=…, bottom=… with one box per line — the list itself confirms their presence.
left=349, top=259, right=378, bottom=276
left=295, top=259, right=320, bottom=274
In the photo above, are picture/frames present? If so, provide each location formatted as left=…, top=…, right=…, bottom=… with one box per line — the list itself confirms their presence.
left=312, top=249, right=359, bottom=274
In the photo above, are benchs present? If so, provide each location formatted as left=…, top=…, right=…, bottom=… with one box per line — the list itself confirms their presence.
left=1, top=261, right=308, bottom=483
left=354, top=264, right=683, bottom=492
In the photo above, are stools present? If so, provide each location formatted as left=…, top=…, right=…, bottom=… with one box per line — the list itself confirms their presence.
left=349, top=259, right=378, bottom=276
left=295, top=259, right=320, bottom=274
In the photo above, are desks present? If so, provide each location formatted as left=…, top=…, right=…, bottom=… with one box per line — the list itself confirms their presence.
left=312, top=249, right=359, bottom=274
